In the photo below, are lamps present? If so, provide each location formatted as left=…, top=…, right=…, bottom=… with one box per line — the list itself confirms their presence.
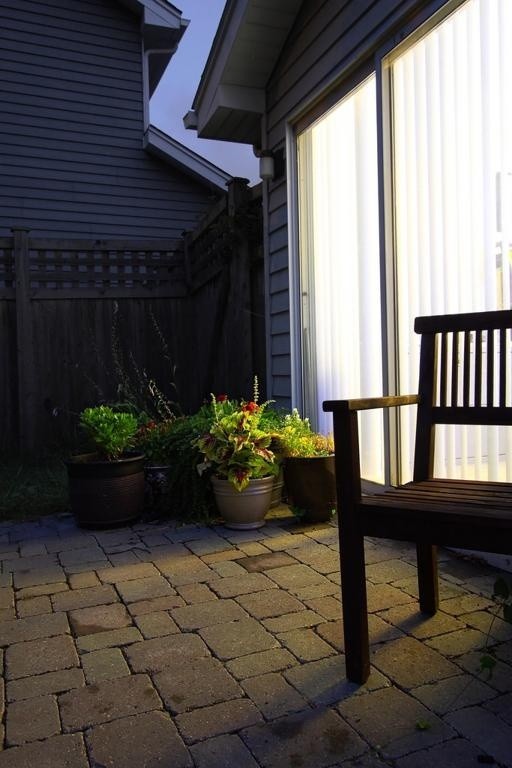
left=259, top=147, right=283, bottom=180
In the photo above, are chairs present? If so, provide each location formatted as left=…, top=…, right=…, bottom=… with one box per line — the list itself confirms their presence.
left=322, top=311, right=512, bottom=685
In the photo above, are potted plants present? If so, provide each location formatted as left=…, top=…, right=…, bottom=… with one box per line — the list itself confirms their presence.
left=71, top=406, right=147, bottom=529
left=264, top=411, right=284, bottom=508
left=180, top=392, right=275, bottom=530
left=139, top=413, right=173, bottom=517
left=281, top=408, right=335, bottom=525
